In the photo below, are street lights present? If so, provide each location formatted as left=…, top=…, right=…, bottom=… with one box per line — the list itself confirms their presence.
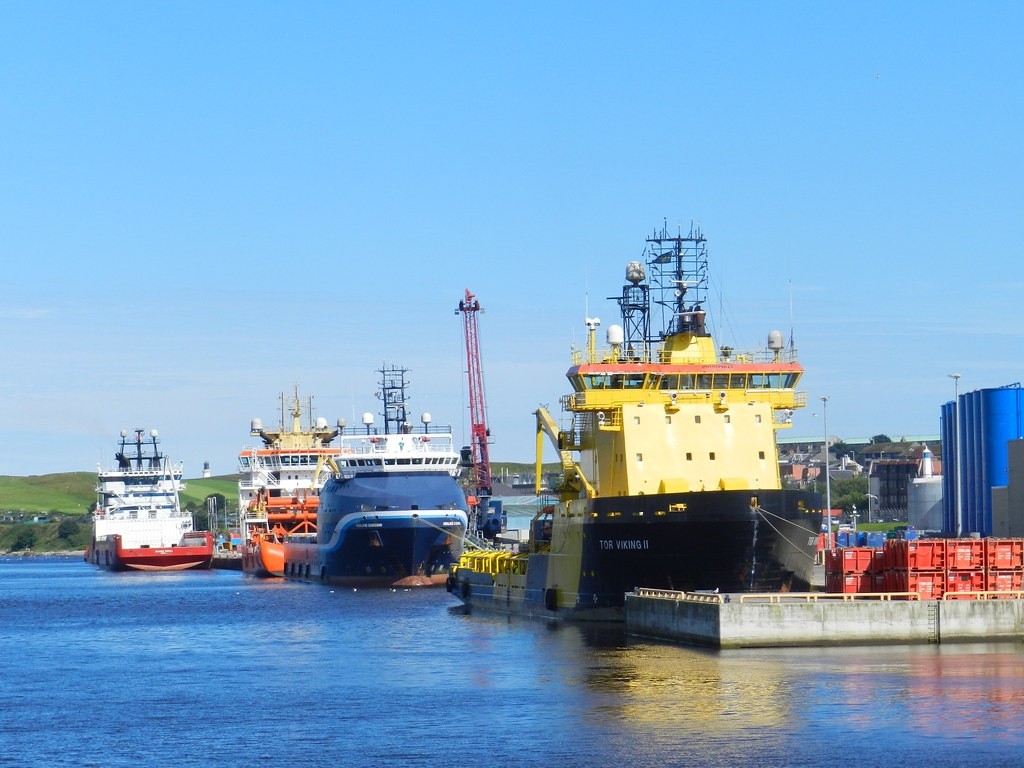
left=820, top=395, right=831, bottom=550
left=948, top=374, right=962, bottom=538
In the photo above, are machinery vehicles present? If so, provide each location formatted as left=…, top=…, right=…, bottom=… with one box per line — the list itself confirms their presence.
left=454, top=288, right=521, bottom=552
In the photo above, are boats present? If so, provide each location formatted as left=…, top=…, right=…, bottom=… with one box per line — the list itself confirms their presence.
left=239, top=384, right=347, bottom=578
left=446, top=216, right=825, bottom=622
left=284, top=362, right=467, bottom=589
left=83, top=428, right=213, bottom=573
